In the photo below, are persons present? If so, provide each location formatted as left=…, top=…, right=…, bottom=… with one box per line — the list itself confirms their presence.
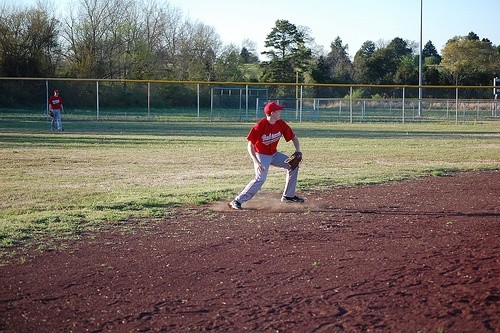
left=226, top=101, right=305, bottom=212
left=48, top=88, right=64, bottom=131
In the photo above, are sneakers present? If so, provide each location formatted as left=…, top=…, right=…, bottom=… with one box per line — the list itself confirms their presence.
left=229, top=200, right=241, bottom=210
left=281, top=195, right=304, bottom=203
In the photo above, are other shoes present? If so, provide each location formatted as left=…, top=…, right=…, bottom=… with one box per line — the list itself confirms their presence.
left=58, top=129, right=64, bottom=131
left=51, top=129, right=54, bottom=132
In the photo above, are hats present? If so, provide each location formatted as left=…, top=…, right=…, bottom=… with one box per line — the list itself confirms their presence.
left=264, top=101, right=286, bottom=114
left=53, top=88, right=60, bottom=93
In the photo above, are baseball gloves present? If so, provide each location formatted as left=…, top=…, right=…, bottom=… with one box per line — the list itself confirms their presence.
left=282, top=150, right=304, bottom=171
left=48, top=110, right=56, bottom=118
